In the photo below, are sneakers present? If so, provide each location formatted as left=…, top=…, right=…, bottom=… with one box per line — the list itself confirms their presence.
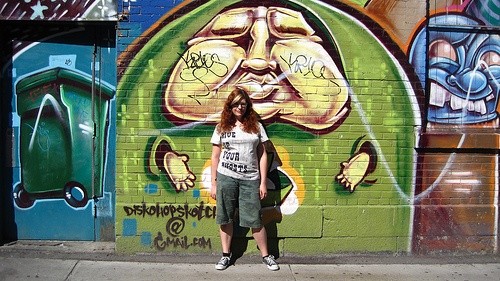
left=215, top=254, right=231, bottom=271
left=263, top=255, right=279, bottom=272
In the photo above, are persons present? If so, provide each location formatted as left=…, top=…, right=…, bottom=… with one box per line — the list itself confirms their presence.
left=209, top=89, right=280, bottom=271
